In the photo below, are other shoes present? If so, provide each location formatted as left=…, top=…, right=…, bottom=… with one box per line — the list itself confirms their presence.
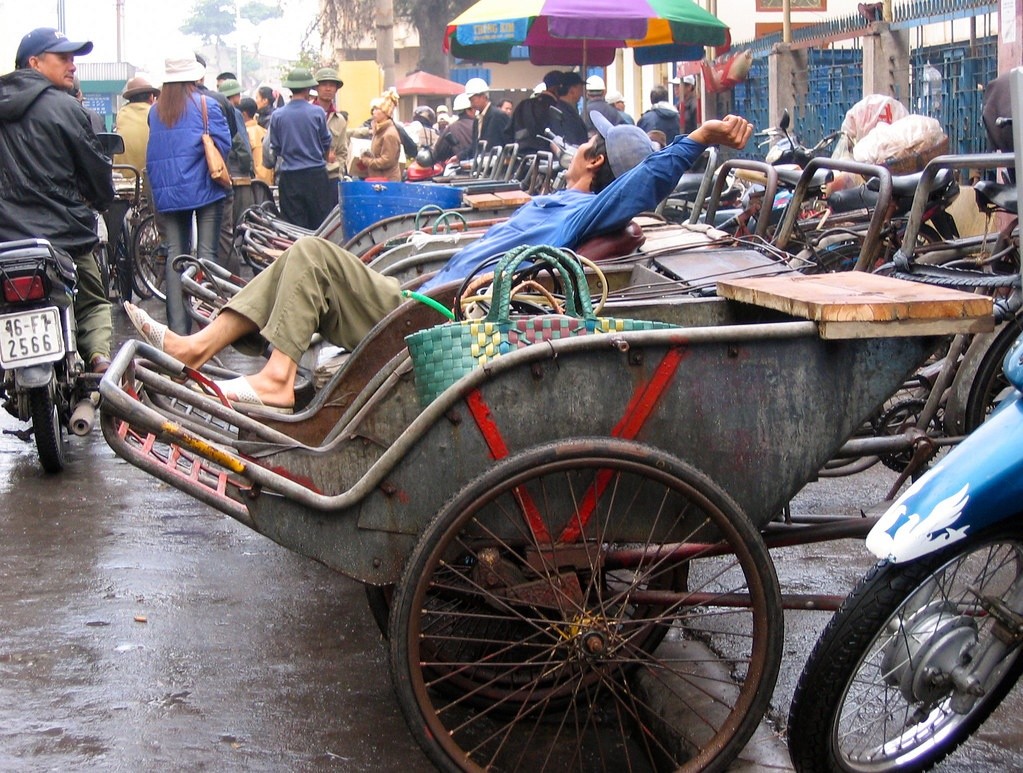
left=89, top=355, right=110, bottom=372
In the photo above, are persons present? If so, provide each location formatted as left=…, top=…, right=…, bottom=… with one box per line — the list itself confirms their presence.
left=66, top=50, right=755, bottom=406
left=0, top=27, right=117, bottom=399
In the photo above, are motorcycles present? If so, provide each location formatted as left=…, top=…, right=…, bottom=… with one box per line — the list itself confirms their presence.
left=656, top=107, right=966, bottom=264
left=786, top=330, right=1023, bottom=773
left=0, top=133, right=125, bottom=474
left=365, top=132, right=469, bottom=183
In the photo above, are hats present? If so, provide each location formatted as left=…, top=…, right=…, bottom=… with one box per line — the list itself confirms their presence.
left=670, top=74, right=695, bottom=86
left=16, top=28, right=93, bottom=70
left=437, top=105, right=448, bottom=112
left=163, top=50, right=206, bottom=83
left=438, top=113, right=449, bottom=123
left=372, top=88, right=400, bottom=118
left=605, top=91, right=627, bottom=104
left=123, top=78, right=161, bottom=99
left=590, top=108, right=654, bottom=180
left=558, top=71, right=589, bottom=85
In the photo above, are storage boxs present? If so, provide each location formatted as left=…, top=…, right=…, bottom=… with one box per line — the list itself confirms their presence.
left=346, top=137, right=406, bottom=177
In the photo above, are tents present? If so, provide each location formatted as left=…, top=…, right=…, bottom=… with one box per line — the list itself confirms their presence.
left=385, top=70, right=471, bottom=118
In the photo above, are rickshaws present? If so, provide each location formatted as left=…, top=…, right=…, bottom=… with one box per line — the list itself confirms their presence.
left=97, top=138, right=1023, bottom=773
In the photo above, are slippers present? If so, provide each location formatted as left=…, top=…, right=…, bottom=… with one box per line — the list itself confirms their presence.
left=122, top=299, right=187, bottom=384
left=190, top=373, right=294, bottom=415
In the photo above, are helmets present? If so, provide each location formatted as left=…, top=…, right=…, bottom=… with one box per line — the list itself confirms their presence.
left=453, top=93, right=472, bottom=111
left=314, top=69, right=344, bottom=90
left=218, top=80, right=246, bottom=99
left=282, top=69, right=319, bottom=88
left=534, top=83, right=547, bottom=94
left=585, top=75, right=605, bottom=91
left=465, top=78, right=490, bottom=94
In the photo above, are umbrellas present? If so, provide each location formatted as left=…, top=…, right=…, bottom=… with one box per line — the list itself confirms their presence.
left=441, top=0, right=734, bottom=126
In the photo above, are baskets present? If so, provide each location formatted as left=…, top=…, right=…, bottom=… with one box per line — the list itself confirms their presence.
left=404, top=241, right=686, bottom=415
left=861, top=133, right=948, bottom=182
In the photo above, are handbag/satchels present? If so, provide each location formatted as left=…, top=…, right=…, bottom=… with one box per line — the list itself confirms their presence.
left=200, top=94, right=233, bottom=188
